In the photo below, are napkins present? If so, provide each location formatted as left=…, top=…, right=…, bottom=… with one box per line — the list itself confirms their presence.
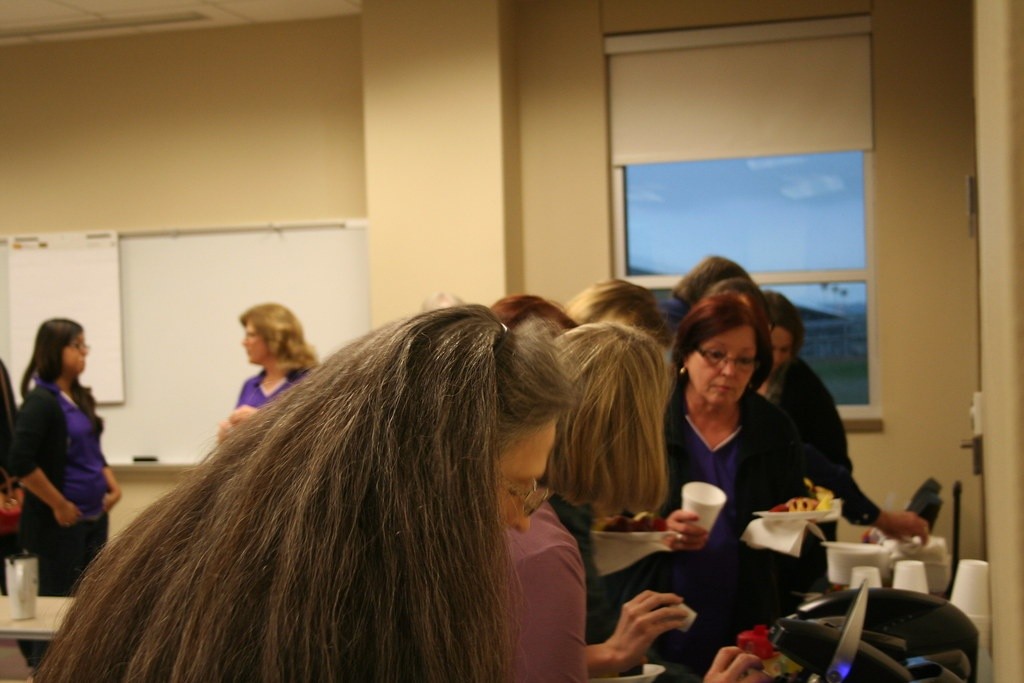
left=741, top=518, right=826, bottom=558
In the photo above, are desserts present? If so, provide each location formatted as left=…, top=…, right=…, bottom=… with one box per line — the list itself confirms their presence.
left=602, top=511, right=668, bottom=533
left=769, top=498, right=818, bottom=512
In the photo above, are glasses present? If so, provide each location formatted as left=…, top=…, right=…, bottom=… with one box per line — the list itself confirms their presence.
left=67, top=342, right=88, bottom=355
left=497, top=473, right=550, bottom=519
left=695, top=345, right=760, bottom=374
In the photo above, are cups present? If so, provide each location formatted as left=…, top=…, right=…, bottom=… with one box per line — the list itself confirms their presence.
left=851, top=567, right=883, bottom=590
left=950, top=558, right=990, bottom=683
left=5, top=556, right=39, bottom=621
left=658, top=599, right=697, bottom=631
left=680, top=482, right=727, bottom=535
left=891, top=560, right=929, bottom=596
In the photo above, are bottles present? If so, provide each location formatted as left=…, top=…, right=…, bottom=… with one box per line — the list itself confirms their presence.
left=737, top=624, right=782, bottom=680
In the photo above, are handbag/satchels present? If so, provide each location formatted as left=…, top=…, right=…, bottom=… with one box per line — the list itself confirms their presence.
left=0, top=471, right=26, bottom=534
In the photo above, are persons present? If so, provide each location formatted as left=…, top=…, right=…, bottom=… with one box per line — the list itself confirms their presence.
left=619, top=290, right=814, bottom=683
left=568, top=278, right=665, bottom=336
left=218, top=304, right=317, bottom=443
left=664, top=256, right=928, bottom=578
left=0, top=317, right=122, bottom=670
left=491, top=295, right=577, bottom=330
left=506, top=323, right=687, bottom=683
left=30, top=304, right=770, bottom=683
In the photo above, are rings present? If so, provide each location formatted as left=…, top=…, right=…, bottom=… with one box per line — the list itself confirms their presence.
left=677, top=534, right=682, bottom=539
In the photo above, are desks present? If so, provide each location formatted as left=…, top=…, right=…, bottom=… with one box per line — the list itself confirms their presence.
left=0, top=596, right=75, bottom=640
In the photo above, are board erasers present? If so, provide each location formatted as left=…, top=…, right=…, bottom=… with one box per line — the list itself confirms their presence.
left=133, top=456, right=158, bottom=463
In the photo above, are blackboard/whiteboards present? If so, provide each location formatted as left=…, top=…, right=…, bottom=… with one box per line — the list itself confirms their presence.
left=0, top=221, right=369, bottom=472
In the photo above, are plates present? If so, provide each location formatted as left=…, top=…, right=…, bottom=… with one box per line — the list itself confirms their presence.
left=821, top=534, right=949, bottom=591
left=587, top=663, right=665, bottom=682
left=590, top=528, right=676, bottom=541
left=753, top=509, right=829, bottom=522
